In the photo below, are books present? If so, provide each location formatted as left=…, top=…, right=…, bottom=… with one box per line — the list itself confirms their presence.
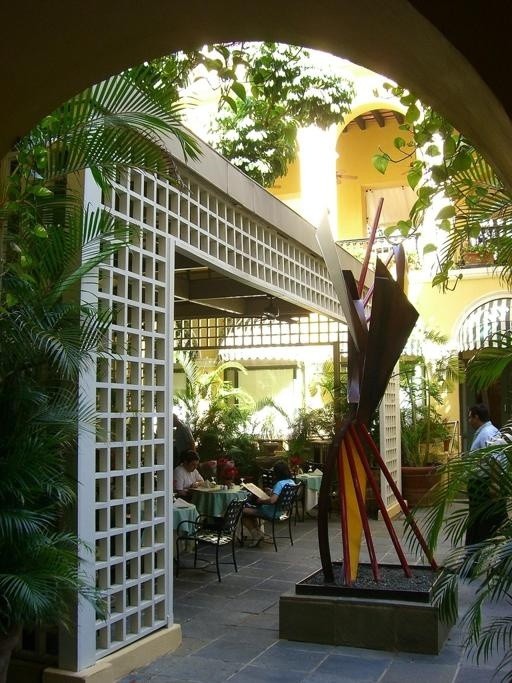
left=183, top=482, right=225, bottom=492
left=240, top=481, right=272, bottom=501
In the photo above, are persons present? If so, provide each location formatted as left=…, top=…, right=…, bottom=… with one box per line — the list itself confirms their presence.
left=439, top=402, right=508, bottom=578
left=241, top=461, right=296, bottom=547
left=174, top=448, right=205, bottom=498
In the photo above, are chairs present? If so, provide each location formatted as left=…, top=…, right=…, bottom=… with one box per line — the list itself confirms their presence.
left=174, top=460, right=330, bottom=583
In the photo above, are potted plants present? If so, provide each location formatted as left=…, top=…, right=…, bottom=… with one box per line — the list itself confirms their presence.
left=459, top=246, right=495, bottom=266
left=392, top=346, right=465, bottom=507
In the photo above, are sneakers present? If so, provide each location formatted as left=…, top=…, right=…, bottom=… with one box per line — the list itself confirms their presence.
left=249, top=531, right=264, bottom=547
